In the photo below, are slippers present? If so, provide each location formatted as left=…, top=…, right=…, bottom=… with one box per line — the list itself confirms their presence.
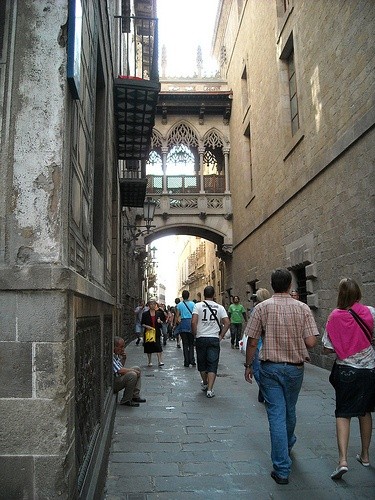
left=355, top=454, right=369, bottom=466
left=330, top=466, right=348, bottom=479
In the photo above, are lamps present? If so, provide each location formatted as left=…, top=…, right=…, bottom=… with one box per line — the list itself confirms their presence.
left=123, top=197, right=157, bottom=244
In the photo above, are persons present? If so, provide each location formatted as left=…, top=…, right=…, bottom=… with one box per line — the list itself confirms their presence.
left=112, top=336, right=146, bottom=407
left=291, top=289, right=299, bottom=300
left=144, top=304, right=150, bottom=311
left=252, top=288, right=271, bottom=403
left=134, top=300, right=148, bottom=346
left=176, top=290, right=195, bottom=367
left=140, top=297, right=164, bottom=367
left=154, top=303, right=176, bottom=345
left=227, top=296, right=247, bottom=349
left=244, top=268, right=320, bottom=484
left=191, top=286, right=230, bottom=398
left=322, top=278, right=375, bottom=479
left=171, top=298, right=181, bottom=348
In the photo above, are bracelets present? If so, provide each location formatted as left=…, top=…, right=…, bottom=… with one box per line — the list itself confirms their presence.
left=244, top=363, right=252, bottom=368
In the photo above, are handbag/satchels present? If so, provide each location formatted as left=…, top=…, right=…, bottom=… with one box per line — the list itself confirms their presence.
left=145, top=327, right=156, bottom=343
left=224, top=328, right=231, bottom=339
left=238, top=340, right=243, bottom=353
left=241, top=333, right=248, bottom=356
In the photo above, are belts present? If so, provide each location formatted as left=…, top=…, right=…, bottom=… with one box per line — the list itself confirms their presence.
left=261, top=359, right=304, bottom=366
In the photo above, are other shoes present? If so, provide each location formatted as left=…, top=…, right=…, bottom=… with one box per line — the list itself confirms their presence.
left=124, top=400, right=139, bottom=407
left=148, top=362, right=153, bottom=366
left=136, top=339, right=141, bottom=344
left=271, top=470, right=288, bottom=484
left=201, top=380, right=209, bottom=389
left=158, top=362, right=164, bottom=366
left=184, top=360, right=196, bottom=367
left=132, top=397, right=146, bottom=403
left=177, top=343, right=181, bottom=348
left=207, top=390, right=215, bottom=397
left=231, top=344, right=239, bottom=349
